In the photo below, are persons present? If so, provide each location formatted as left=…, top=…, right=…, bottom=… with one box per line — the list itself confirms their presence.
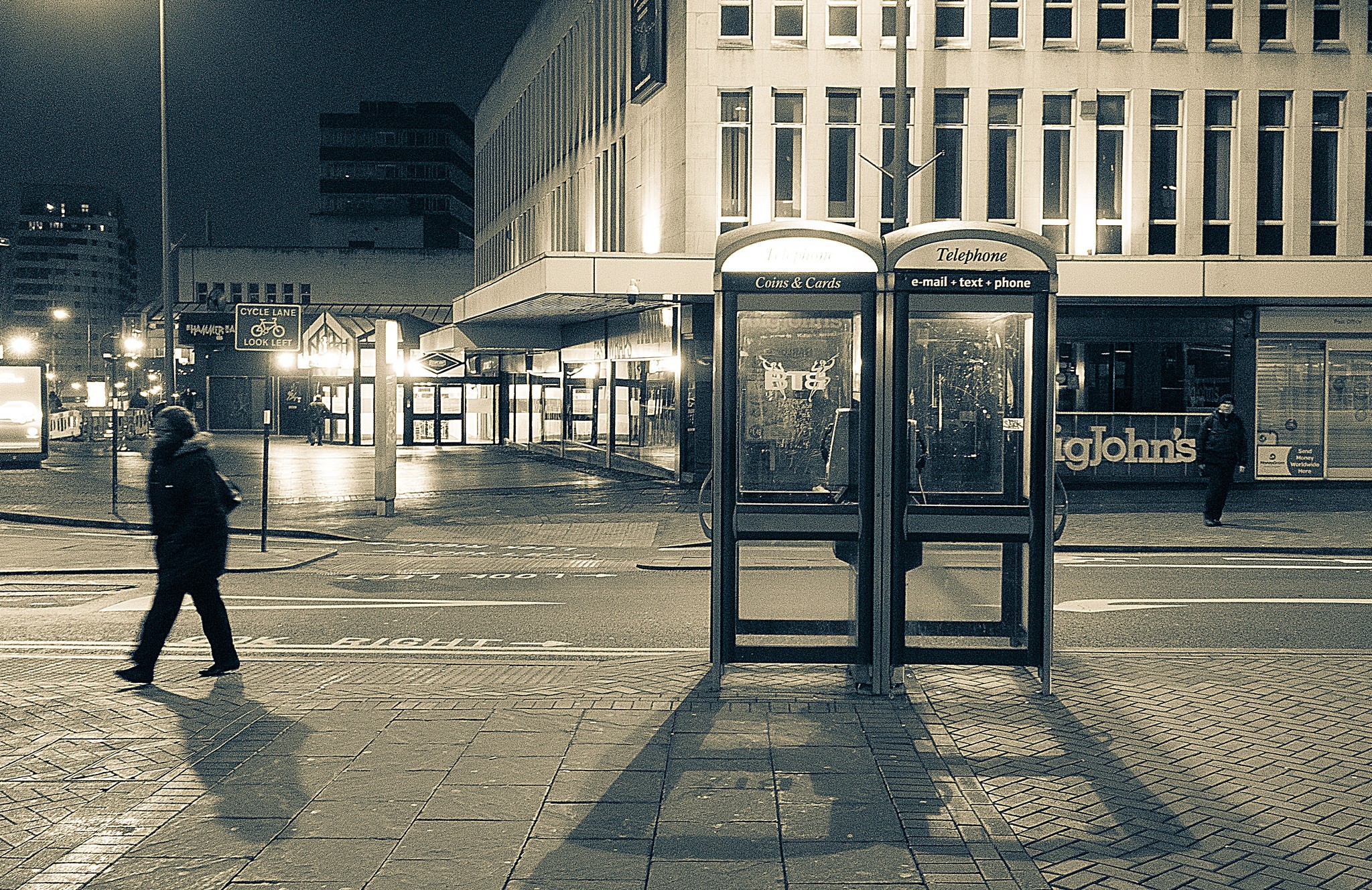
left=307, top=394, right=330, bottom=446
left=130, top=388, right=148, bottom=408
left=115, top=405, right=240, bottom=684
left=48, top=391, right=58, bottom=413
left=1196, top=394, right=1248, bottom=527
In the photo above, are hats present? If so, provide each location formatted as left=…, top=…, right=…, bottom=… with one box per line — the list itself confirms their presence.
left=1220, top=394, right=1235, bottom=405
left=159, top=405, right=198, bottom=438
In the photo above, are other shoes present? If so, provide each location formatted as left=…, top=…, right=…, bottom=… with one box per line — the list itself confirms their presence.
left=199, top=657, right=240, bottom=675
left=116, top=664, right=153, bottom=684
left=1203, top=516, right=1223, bottom=527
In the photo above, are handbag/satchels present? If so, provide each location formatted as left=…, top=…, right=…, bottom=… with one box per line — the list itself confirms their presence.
left=214, top=470, right=242, bottom=515
